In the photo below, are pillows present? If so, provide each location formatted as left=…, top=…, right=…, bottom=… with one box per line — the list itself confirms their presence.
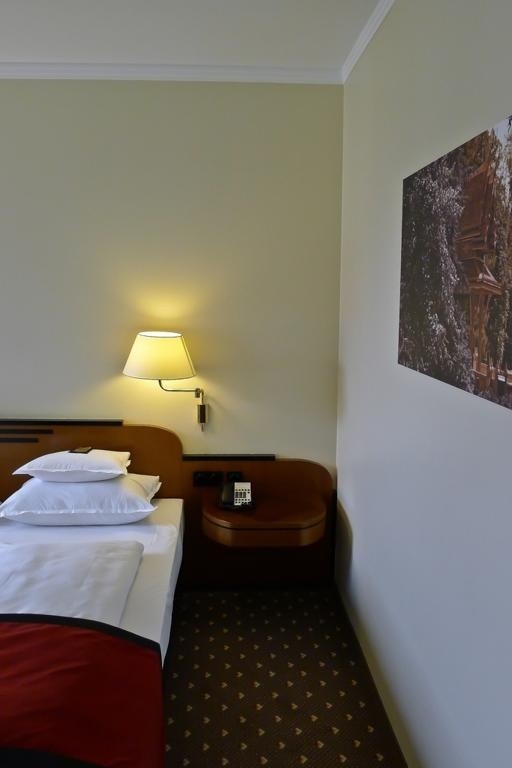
left=10, top=448, right=131, bottom=483
left=0, top=473, right=163, bottom=526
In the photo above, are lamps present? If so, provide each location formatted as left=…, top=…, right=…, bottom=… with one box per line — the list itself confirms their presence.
left=122, top=330, right=207, bottom=432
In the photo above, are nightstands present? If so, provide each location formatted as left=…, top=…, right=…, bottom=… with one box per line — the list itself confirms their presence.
left=186, top=459, right=335, bottom=548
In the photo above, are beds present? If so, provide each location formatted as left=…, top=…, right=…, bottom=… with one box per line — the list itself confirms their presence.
left=0, top=419, right=186, bottom=768
